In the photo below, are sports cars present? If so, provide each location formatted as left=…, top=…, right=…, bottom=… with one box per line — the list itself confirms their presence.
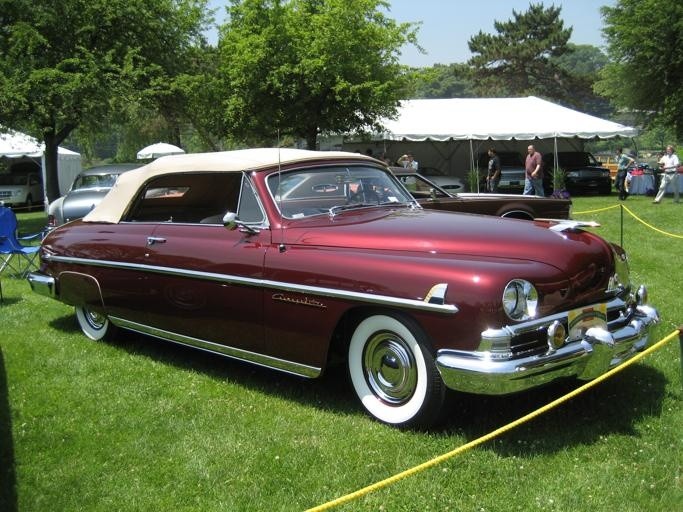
left=277, top=166, right=571, bottom=225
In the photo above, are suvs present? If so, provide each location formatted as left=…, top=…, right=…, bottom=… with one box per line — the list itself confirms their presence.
left=475, top=152, right=526, bottom=191
left=0, top=170, right=40, bottom=214
left=541, top=151, right=611, bottom=197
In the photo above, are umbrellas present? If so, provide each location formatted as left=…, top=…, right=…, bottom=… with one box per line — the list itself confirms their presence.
left=137, top=141, right=186, bottom=160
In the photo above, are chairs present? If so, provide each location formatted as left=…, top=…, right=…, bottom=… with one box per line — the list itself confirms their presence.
left=0, top=205, right=50, bottom=285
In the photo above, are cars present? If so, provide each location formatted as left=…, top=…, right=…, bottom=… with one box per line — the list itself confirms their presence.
left=412, top=167, right=465, bottom=195
left=47, top=163, right=167, bottom=229
left=24, top=144, right=657, bottom=433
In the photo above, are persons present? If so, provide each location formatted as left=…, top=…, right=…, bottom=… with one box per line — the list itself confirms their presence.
left=486, top=148, right=502, bottom=193
left=366, top=149, right=374, bottom=157
left=652, top=144, right=680, bottom=204
left=396, top=152, right=419, bottom=190
left=614, top=147, right=634, bottom=200
left=356, top=149, right=361, bottom=153
left=523, top=144, right=545, bottom=197
